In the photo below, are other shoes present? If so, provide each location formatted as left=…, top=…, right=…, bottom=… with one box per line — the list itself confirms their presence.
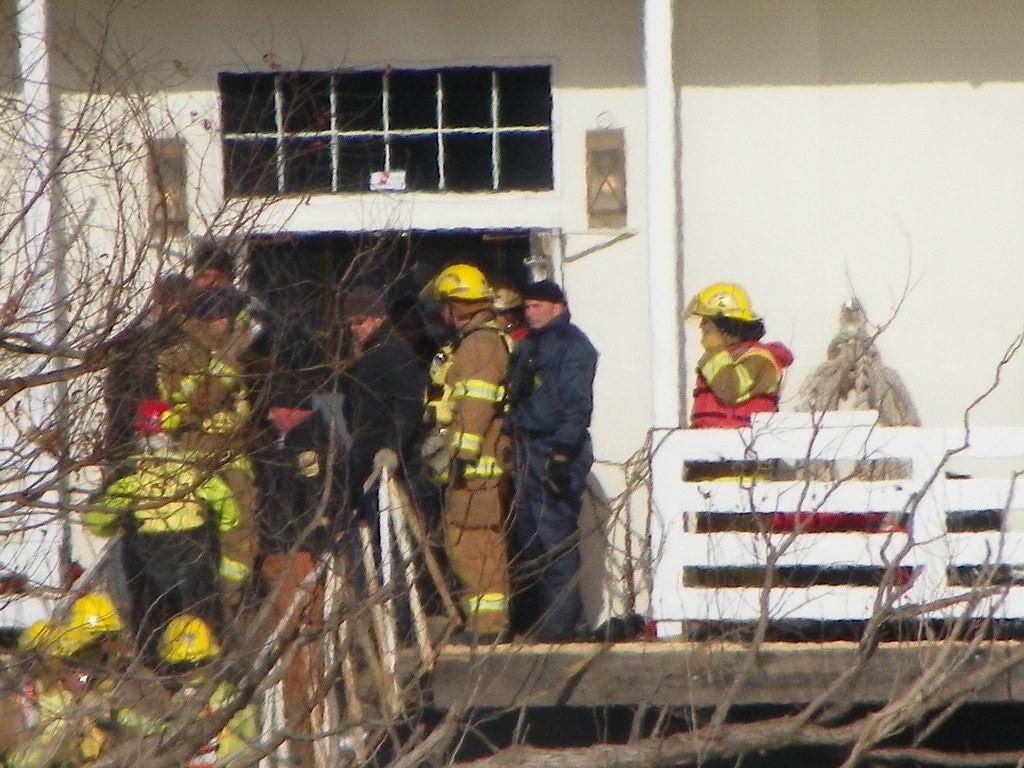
left=448, top=631, right=512, bottom=646
left=230, top=585, right=267, bottom=614
left=515, top=618, right=592, bottom=644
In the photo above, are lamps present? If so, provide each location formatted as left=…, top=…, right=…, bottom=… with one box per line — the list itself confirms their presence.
left=145, top=139, right=190, bottom=237
left=586, top=128, right=628, bottom=231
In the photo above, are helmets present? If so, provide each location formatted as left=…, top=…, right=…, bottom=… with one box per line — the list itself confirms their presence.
left=68, top=593, right=124, bottom=633
left=18, top=619, right=82, bottom=656
left=130, top=401, right=172, bottom=432
left=691, top=283, right=762, bottom=323
left=157, top=614, right=219, bottom=664
left=435, top=262, right=495, bottom=304
left=487, top=285, right=523, bottom=309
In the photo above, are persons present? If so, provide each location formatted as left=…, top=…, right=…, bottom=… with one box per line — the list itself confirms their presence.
left=0, top=593, right=256, bottom=768
left=686, top=281, right=794, bottom=643
left=501, top=280, right=599, bottom=644
left=81, top=249, right=265, bottom=692
left=423, top=264, right=530, bottom=647
left=344, top=284, right=440, bottom=642
left=254, top=381, right=346, bottom=768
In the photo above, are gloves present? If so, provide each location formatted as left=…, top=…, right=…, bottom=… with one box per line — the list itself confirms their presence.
left=541, top=446, right=576, bottom=501
left=699, top=316, right=722, bottom=349
left=447, top=457, right=466, bottom=490
left=371, top=447, right=401, bottom=482
left=297, top=450, right=320, bottom=478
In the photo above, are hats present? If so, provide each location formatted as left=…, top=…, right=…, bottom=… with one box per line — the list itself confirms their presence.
left=522, top=279, right=565, bottom=304
left=269, top=375, right=314, bottom=409
